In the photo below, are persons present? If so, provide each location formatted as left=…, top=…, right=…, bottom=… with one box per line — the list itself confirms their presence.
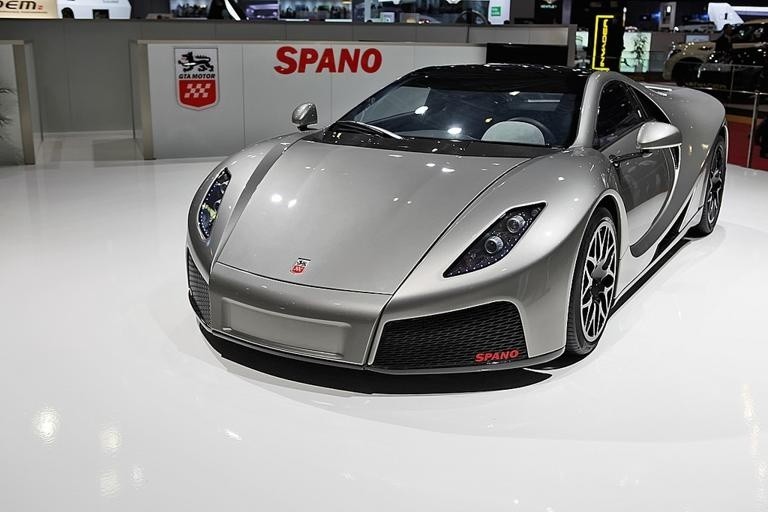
left=165, top=0, right=382, bottom=21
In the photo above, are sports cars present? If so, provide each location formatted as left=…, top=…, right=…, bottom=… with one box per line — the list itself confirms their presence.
left=184, top=63, right=729, bottom=376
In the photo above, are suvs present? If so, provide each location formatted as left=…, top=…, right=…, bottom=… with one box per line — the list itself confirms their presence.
left=662, top=19, right=767, bottom=89
